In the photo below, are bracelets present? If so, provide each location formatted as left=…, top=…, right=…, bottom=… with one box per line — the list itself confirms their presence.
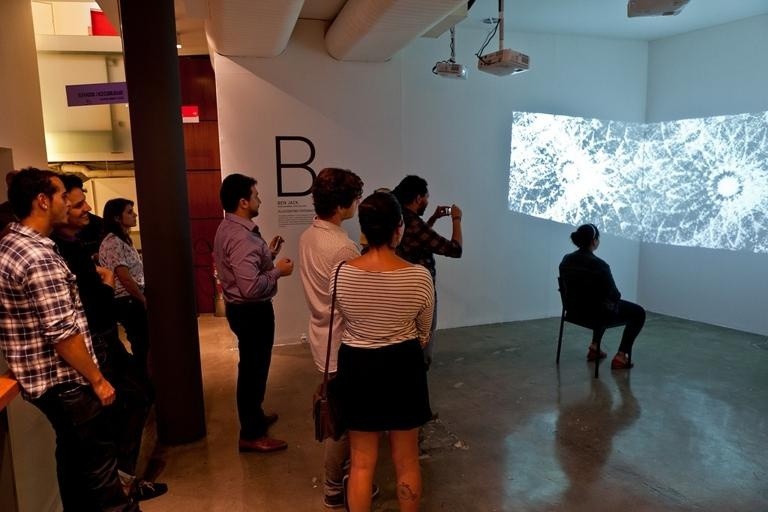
left=450, top=216, right=462, bottom=220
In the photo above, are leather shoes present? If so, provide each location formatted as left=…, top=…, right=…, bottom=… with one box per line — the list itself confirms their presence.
left=587, top=347, right=608, bottom=361
left=126, top=478, right=169, bottom=501
left=237, top=437, right=288, bottom=455
left=259, top=410, right=280, bottom=430
left=612, top=357, right=634, bottom=370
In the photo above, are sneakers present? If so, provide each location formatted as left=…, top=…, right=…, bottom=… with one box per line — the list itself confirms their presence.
left=325, top=477, right=381, bottom=508
left=342, top=456, right=355, bottom=471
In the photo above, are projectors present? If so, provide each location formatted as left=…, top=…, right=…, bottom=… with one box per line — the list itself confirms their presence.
left=626, top=0, right=689, bottom=19
left=472, top=50, right=532, bottom=79
left=425, top=59, right=468, bottom=82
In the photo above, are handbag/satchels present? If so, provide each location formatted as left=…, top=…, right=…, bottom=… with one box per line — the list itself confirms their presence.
left=312, top=377, right=343, bottom=445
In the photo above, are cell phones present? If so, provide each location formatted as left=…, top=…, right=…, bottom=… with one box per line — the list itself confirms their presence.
left=442, top=206, right=457, bottom=216
left=271, top=237, right=286, bottom=250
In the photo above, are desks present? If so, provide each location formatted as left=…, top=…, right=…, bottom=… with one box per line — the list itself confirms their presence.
left=0, top=376, right=19, bottom=512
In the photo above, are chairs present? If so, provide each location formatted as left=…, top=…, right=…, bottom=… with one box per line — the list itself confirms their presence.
left=557, top=276, right=632, bottom=376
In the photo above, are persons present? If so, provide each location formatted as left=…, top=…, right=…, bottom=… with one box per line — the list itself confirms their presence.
left=98, top=199, right=147, bottom=357
left=214, top=173, right=295, bottom=453
left=393, top=174, right=462, bottom=367
left=300, top=167, right=381, bottom=506
left=0, top=168, right=139, bottom=512
left=558, top=224, right=646, bottom=370
left=329, top=188, right=436, bottom=512
left=359, top=187, right=392, bottom=251
left=49, top=174, right=168, bottom=502
